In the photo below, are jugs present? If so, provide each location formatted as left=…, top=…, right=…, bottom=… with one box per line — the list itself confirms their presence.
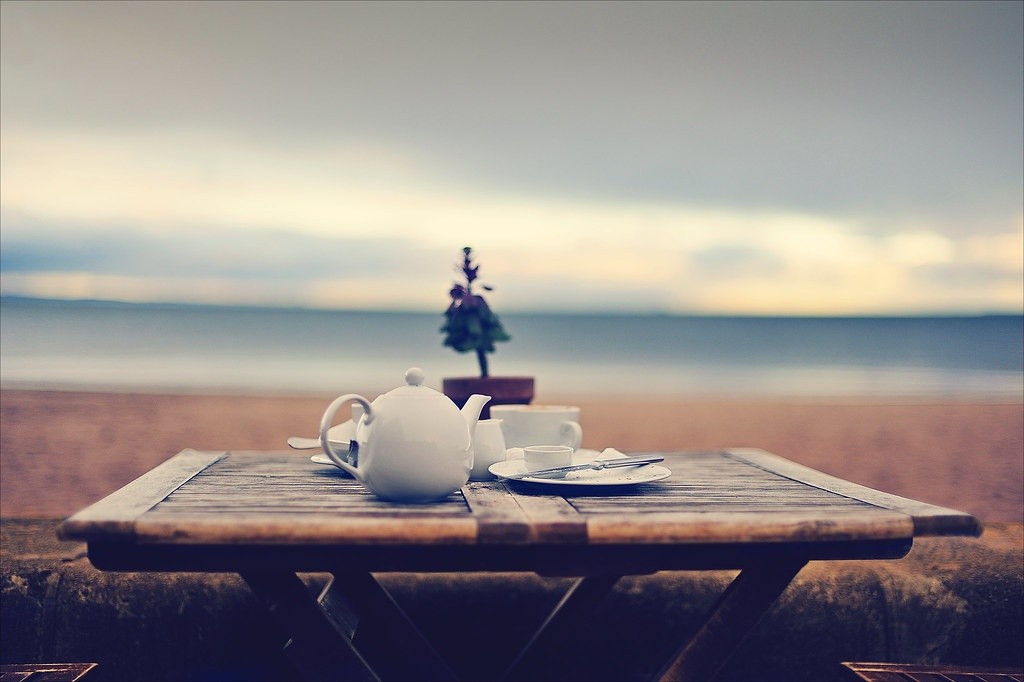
left=468, top=419, right=508, bottom=482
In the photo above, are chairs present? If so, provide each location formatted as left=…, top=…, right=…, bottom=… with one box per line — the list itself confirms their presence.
left=0, top=662, right=101, bottom=682
left=840, top=660, right=1024, bottom=682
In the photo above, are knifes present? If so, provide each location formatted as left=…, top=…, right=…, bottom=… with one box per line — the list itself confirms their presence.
left=496, top=454, right=665, bottom=483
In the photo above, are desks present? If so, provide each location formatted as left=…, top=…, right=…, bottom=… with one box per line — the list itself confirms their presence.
left=55, top=446, right=985, bottom=682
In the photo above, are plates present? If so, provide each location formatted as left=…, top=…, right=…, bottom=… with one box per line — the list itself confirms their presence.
left=488, top=456, right=672, bottom=494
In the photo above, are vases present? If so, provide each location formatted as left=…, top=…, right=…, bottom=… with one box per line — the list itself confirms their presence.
left=443, top=376, right=536, bottom=421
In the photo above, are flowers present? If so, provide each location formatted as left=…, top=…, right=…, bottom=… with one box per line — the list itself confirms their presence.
left=438, top=245, right=513, bottom=376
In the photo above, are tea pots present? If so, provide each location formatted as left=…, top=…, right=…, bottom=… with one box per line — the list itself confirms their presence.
left=317, top=367, right=493, bottom=505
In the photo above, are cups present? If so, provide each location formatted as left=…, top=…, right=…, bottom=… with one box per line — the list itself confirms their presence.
left=351, top=403, right=371, bottom=433
left=490, top=404, right=583, bottom=454
left=524, top=445, right=574, bottom=479
